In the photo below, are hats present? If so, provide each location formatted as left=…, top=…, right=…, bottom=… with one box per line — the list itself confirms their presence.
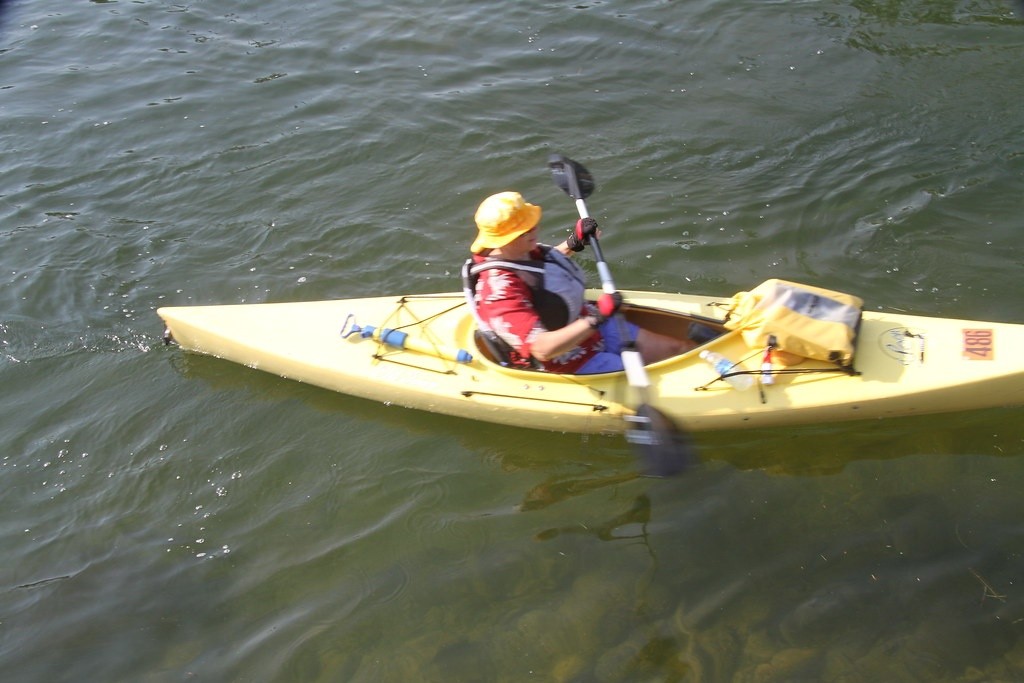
left=469, top=191, right=542, bottom=254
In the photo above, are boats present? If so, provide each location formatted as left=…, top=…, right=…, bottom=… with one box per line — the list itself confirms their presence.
left=156, top=288, right=1023, bottom=437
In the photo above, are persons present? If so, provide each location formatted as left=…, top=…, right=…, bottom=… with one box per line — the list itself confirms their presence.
left=470, top=191, right=699, bottom=374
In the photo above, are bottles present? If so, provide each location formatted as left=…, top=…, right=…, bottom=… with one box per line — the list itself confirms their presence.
left=699, top=350, right=754, bottom=393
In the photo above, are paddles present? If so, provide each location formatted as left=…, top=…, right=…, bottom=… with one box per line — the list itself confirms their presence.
left=548, top=152, right=684, bottom=474
